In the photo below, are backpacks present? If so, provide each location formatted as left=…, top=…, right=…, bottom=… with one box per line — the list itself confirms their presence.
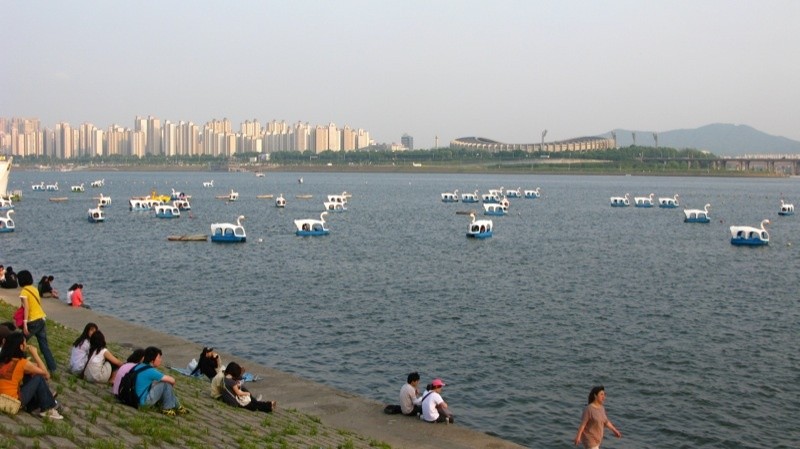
left=118, top=366, right=155, bottom=408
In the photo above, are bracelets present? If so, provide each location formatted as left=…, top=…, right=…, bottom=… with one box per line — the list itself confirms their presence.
left=24, top=320, right=28, bottom=322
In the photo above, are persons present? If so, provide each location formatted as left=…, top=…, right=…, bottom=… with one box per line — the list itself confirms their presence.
left=18, top=269, right=56, bottom=370
left=0, top=321, right=65, bottom=420
left=400, top=372, right=454, bottom=424
left=302, top=224, right=307, bottom=230
left=66, top=282, right=92, bottom=309
left=190, top=346, right=277, bottom=414
left=575, top=386, right=621, bottom=449
left=0, top=263, right=18, bottom=289
left=473, top=226, right=485, bottom=232
left=38, top=275, right=59, bottom=299
left=70, top=322, right=189, bottom=416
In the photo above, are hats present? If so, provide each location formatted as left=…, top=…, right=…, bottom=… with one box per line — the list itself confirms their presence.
left=432, top=379, right=445, bottom=386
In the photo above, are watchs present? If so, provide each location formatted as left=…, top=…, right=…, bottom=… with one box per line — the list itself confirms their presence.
left=215, top=355, right=218, bottom=359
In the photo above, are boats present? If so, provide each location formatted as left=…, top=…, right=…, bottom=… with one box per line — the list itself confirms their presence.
left=0, top=194, right=15, bottom=211
left=210, top=215, right=247, bottom=243
left=47, top=182, right=59, bottom=192
left=323, top=191, right=353, bottom=213
left=609, top=193, right=630, bottom=207
left=633, top=192, right=654, bottom=208
left=0, top=209, right=16, bottom=233
left=229, top=189, right=237, bottom=201
left=441, top=185, right=542, bottom=215
left=97, top=192, right=112, bottom=207
left=274, top=193, right=287, bottom=208
left=91, top=177, right=106, bottom=189
left=128, top=187, right=191, bottom=220
left=658, top=194, right=680, bottom=208
left=88, top=202, right=105, bottom=222
left=730, top=218, right=770, bottom=245
left=30, top=181, right=45, bottom=192
left=202, top=179, right=214, bottom=189
left=683, top=204, right=714, bottom=223
left=778, top=199, right=795, bottom=216
left=71, top=183, right=85, bottom=193
left=466, top=212, right=492, bottom=239
left=293, top=211, right=331, bottom=237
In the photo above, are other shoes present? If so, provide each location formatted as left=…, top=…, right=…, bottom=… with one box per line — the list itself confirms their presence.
left=40, top=409, right=64, bottom=419
left=55, top=402, right=61, bottom=409
left=271, top=401, right=277, bottom=410
left=256, top=394, right=262, bottom=401
left=178, top=406, right=189, bottom=414
left=51, top=390, right=57, bottom=399
left=160, top=408, right=177, bottom=417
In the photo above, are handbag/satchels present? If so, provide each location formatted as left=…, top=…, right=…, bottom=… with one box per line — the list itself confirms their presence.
left=14, top=306, right=25, bottom=327
left=235, top=394, right=251, bottom=407
left=0, top=394, right=21, bottom=415
